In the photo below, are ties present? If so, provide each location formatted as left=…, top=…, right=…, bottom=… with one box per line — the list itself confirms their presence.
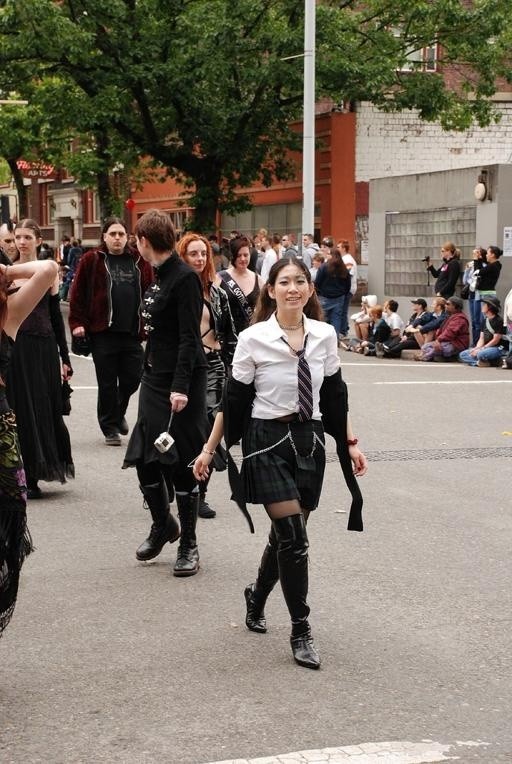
left=280, top=334, right=313, bottom=423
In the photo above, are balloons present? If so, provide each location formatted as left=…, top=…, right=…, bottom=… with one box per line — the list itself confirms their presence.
left=126, top=199, right=135, bottom=209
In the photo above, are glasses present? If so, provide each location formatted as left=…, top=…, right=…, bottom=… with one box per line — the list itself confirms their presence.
left=110, top=232, right=124, bottom=236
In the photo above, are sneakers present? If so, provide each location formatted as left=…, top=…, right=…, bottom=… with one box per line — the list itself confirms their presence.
left=200, top=498, right=215, bottom=518
left=346, top=345, right=390, bottom=356
left=477, top=360, right=490, bottom=367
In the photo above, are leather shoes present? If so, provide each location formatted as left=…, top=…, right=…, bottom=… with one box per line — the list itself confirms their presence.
left=118, top=417, right=128, bottom=435
left=105, top=435, right=121, bottom=445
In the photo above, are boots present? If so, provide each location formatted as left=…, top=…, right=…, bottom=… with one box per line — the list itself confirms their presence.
left=137, top=480, right=180, bottom=561
left=244, top=512, right=307, bottom=633
left=173, top=493, right=199, bottom=577
left=272, top=512, right=320, bottom=669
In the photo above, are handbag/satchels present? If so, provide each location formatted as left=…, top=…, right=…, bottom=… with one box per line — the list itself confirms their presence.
left=71, top=333, right=90, bottom=356
left=461, top=284, right=470, bottom=299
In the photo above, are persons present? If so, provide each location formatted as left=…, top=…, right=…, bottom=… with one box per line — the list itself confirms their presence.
left=190, top=254, right=368, bottom=671
left=119, top=210, right=211, bottom=580
left=1, top=254, right=61, bottom=641
left=2, top=208, right=370, bottom=519
left=368, top=240, right=512, bottom=370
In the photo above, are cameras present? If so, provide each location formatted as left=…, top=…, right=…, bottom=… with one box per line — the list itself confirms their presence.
left=154, top=431, right=174, bottom=452
left=422, top=255, right=429, bottom=262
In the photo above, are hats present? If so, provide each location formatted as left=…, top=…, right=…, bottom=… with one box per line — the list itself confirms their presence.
left=449, top=296, right=463, bottom=308
left=411, top=299, right=427, bottom=305
left=481, top=297, right=500, bottom=309
